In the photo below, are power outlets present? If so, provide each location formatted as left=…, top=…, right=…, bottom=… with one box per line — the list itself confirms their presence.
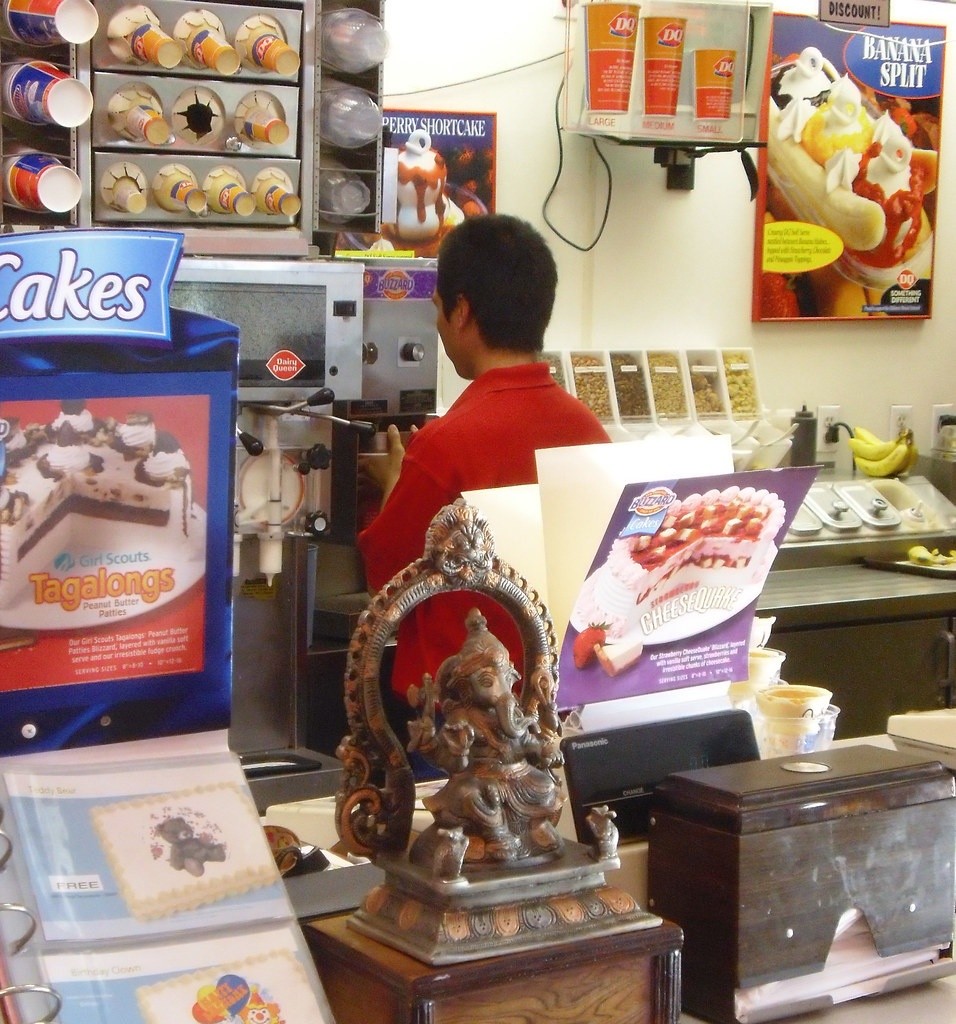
left=890, top=406, right=912, bottom=441
left=817, top=406, right=840, bottom=451
left=932, top=404, right=952, bottom=448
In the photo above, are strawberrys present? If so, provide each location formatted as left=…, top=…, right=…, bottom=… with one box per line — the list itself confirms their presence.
left=572, top=622, right=611, bottom=669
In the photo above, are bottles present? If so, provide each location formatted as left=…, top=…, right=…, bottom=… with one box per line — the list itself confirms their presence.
left=790, top=403, right=816, bottom=467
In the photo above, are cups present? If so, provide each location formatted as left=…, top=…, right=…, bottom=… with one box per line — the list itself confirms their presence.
left=153, top=171, right=207, bottom=214
left=0, top=140, right=82, bottom=213
left=107, top=176, right=146, bottom=213
left=643, top=17, right=687, bottom=117
left=318, top=160, right=370, bottom=223
left=739, top=699, right=841, bottom=760
left=748, top=646, right=786, bottom=693
left=180, top=20, right=240, bottom=76
left=208, top=173, right=255, bottom=217
left=119, top=98, right=169, bottom=145
left=308, top=543, right=319, bottom=646
left=256, top=179, right=301, bottom=217
left=586, top=2, right=641, bottom=115
left=240, top=24, right=300, bottom=76
left=695, top=49, right=737, bottom=119
left=0, top=57, right=92, bottom=127
left=2, top=0, right=99, bottom=54
left=320, top=78, right=381, bottom=146
left=319, top=9, right=388, bottom=72
left=242, top=102, right=290, bottom=146
left=124, top=18, right=181, bottom=68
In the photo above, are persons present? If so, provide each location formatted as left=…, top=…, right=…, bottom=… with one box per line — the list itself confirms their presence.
left=358, top=214, right=613, bottom=785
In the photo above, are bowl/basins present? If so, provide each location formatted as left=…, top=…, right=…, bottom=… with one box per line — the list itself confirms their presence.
left=342, top=184, right=487, bottom=250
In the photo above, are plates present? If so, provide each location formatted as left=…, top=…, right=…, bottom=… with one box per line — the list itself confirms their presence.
left=569, top=537, right=778, bottom=644
left=0, top=504, right=206, bottom=628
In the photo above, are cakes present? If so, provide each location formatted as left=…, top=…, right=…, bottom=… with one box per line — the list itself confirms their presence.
left=593, top=485, right=787, bottom=636
left=91, top=779, right=277, bottom=919
left=134, top=949, right=325, bottom=1024
left=0, top=399, right=193, bottom=607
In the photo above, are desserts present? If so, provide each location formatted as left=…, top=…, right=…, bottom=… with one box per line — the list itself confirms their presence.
left=592, top=636, right=644, bottom=677
left=358, top=128, right=464, bottom=259
left=768, top=46, right=939, bottom=272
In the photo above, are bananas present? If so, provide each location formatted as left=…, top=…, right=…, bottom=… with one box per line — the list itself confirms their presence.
left=849, top=425, right=918, bottom=477
left=907, top=545, right=949, bottom=565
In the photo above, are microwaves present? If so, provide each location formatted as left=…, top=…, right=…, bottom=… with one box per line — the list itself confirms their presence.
left=170, top=255, right=364, bottom=402
